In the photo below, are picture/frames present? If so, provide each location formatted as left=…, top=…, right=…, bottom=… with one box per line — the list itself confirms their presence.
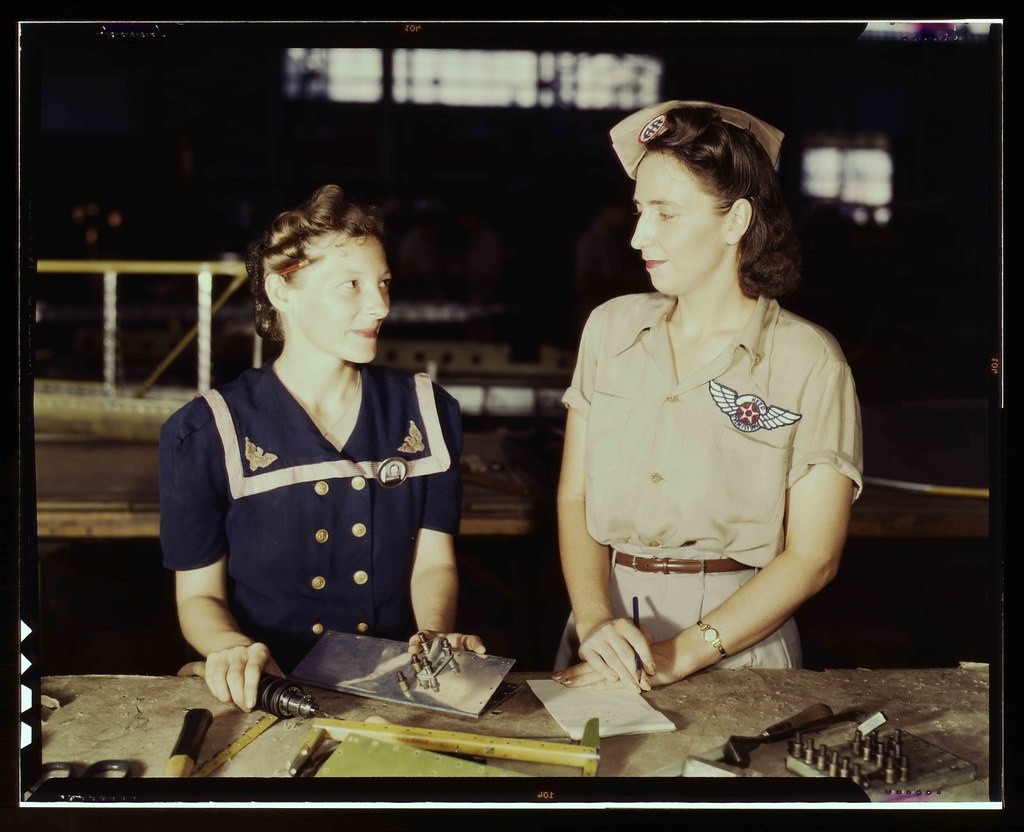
left=0, top=0, right=1024, bottom=832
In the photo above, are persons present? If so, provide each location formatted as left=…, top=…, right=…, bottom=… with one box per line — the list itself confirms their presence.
left=159, top=185, right=485, bottom=715
left=557, top=101, right=860, bottom=692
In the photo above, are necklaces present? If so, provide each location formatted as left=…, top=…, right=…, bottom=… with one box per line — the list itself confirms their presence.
left=300, top=370, right=360, bottom=437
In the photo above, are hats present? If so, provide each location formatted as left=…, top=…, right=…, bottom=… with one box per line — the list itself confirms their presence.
left=610, top=100, right=784, bottom=180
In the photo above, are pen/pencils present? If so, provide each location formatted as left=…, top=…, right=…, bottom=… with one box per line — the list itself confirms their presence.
left=632, top=595, right=643, bottom=681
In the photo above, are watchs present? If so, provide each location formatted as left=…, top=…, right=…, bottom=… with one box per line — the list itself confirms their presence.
left=698, top=620, right=726, bottom=659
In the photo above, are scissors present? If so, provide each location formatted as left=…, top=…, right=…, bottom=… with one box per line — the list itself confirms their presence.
left=27, top=759, right=132, bottom=791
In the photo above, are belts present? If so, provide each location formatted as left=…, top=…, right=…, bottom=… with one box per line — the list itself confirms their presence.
left=615, top=550, right=760, bottom=575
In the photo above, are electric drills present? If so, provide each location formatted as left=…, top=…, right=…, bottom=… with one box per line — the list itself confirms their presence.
left=252, top=672, right=345, bottom=722
